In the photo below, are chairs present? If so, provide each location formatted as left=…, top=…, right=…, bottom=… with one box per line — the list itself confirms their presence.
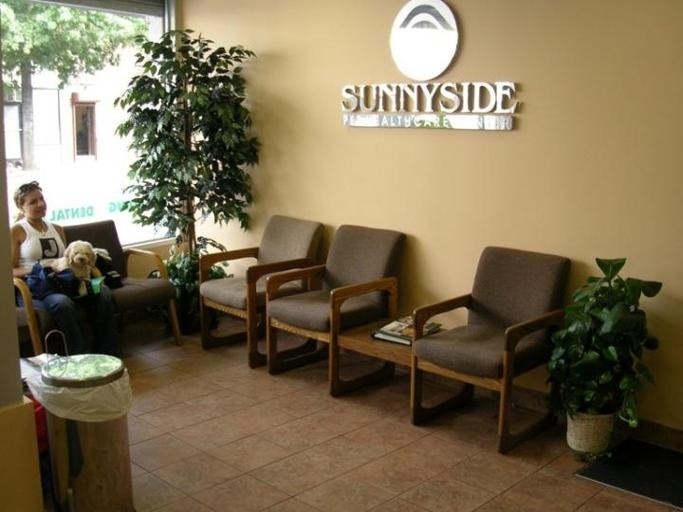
left=266, top=224, right=404, bottom=396
left=197, top=215, right=324, bottom=369
left=411, top=245, right=570, bottom=453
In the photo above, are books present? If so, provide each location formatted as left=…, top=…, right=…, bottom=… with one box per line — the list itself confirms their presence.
left=371, top=313, right=443, bottom=346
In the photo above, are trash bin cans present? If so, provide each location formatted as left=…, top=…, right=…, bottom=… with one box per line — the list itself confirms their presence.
left=41, top=354, right=134, bottom=512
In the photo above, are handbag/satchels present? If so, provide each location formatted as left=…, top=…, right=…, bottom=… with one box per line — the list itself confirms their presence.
left=26, top=258, right=80, bottom=302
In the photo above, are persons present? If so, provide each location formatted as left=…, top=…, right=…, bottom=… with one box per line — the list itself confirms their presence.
left=8, top=179, right=116, bottom=357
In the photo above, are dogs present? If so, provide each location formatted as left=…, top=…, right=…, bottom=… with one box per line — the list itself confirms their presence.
left=50, top=240, right=105, bottom=298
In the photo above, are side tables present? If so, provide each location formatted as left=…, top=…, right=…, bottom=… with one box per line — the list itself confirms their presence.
left=339, top=316, right=449, bottom=370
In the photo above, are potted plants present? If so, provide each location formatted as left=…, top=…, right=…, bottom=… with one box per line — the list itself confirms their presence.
left=546, top=257, right=662, bottom=455
left=114, top=29, right=260, bottom=333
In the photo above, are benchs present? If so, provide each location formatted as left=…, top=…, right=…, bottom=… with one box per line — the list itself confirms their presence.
left=10, top=221, right=184, bottom=373
